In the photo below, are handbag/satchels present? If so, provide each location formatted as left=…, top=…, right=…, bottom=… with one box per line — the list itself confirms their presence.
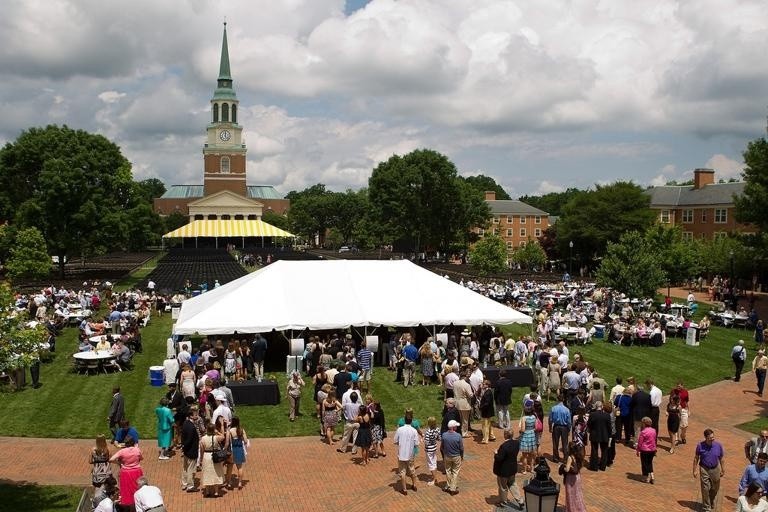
left=534, top=413, right=544, bottom=432
left=211, top=434, right=227, bottom=464
left=226, top=451, right=234, bottom=464
left=613, top=407, right=620, bottom=417
left=732, top=351, right=741, bottom=362
left=566, top=456, right=577, bottom=476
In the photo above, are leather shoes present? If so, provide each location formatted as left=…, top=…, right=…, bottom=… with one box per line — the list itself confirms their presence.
left=399, top=489, right=407, bottom=495
left=336, top=449, right=344, bottom=453
left=450, top=490, right=458, bottom=496
left=187, top=488, right=199, bottom=492
left=411, top=486, right=417, bottom=492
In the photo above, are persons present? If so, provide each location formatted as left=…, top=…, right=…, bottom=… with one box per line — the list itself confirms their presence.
left=6, top=278, right=172, bottom=375
left=114, top=417, right=139, bottom=449
left=693, top=428, right=725, bottom=510
left=731, top=339, right=747, bottom=382
left=233, top=253, right=274, bottom=268
left=285, top=324, right=691, bottom=511
left=735, top=430, right=767, bottom=512
left=88, top=433, right=166, bottom=511
left=410, top=254, right=768, bottom=347
left=155, top=333, right=267, bottom=497
left=177, top=279, right=221, bottom=301
left=106, top=385, right=126, bottom=444
left=751, top=345, right=767, bottom=397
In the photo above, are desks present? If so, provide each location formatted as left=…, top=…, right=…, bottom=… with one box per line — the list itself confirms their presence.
left=220, top=381, right=282, bottom=405
left=479, top=364, right=534, bottom=387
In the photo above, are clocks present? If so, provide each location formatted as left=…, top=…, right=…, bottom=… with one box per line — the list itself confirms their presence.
left=219, top=129, right=231, bottom=142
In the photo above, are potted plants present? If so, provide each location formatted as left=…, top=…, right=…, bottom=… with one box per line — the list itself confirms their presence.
left=0, top=279, right=52, bottom=390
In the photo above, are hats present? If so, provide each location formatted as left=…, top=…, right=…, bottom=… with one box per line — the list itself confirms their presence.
left=215, top=395, right=227, bottom=402
left=552, top=355, right=559, bottom=364
left=196, top=357, right=205, bottom=367
left=448, top=419, right=460, bottom=427
left=756, top=350, right=763, bottom=354
left=461, top=328, right=471, bottom=336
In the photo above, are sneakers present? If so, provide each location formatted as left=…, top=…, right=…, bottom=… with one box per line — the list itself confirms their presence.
left=159, top=455, right=170, bottom=460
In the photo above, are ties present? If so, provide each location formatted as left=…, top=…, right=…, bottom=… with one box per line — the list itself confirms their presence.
left=758, top=442, right=763, bottom=454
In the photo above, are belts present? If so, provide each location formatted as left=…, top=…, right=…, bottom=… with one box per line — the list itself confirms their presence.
left=144, top=504, right=163, bottom=512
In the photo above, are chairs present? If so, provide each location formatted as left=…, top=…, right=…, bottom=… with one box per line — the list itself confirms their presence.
left=18, top=246, right=247, bottom=375
left=424, top=260, right=752, bottom=345
